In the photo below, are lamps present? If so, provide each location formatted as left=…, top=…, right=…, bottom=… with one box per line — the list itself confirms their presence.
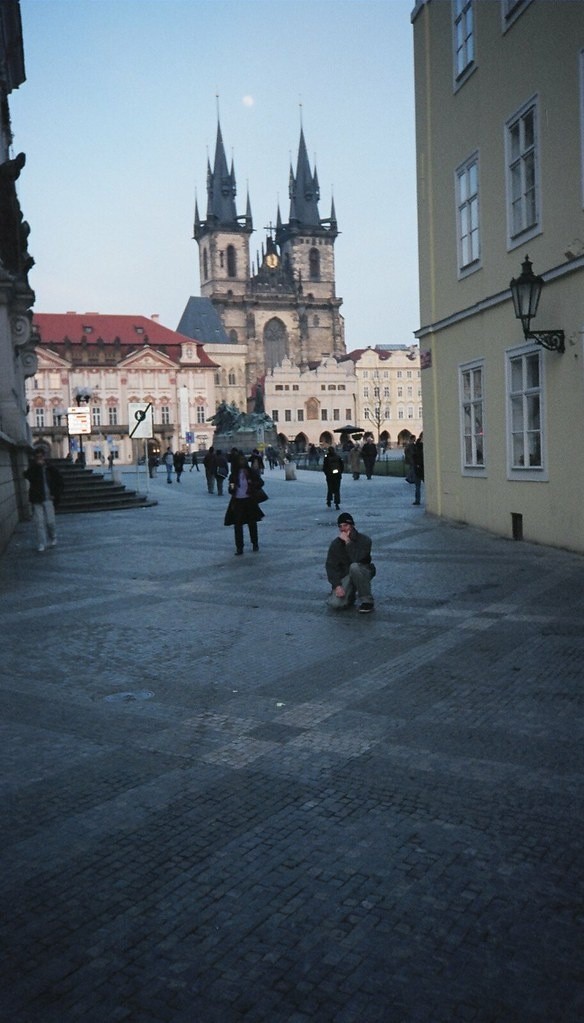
left=510, top=254, right=565, bottom=354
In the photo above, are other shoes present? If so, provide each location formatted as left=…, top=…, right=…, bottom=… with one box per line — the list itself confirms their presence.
left=412, top=502, right=420, bottom=505
left=38, top=544, right=44, bottom=551
left=253, top=544, right=258, bottom=551
left=235, top=551, right=243, bottom=555
left=359, top=603, right=373, bottom=612
left=52, top=538, right=56, bottom=545
left=336, top=507, right=339, bottom=510
left=327, top=501, right=331, bottom=507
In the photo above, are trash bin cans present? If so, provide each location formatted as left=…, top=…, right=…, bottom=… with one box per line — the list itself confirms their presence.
left=285, top=462, right=297, bottom=480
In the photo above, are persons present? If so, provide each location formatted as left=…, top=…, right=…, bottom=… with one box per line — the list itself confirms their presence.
left=24, top=448, right=61, bottom=552
left=225, top=450, right=263, bottom=556
left=325, top=513, right=376, bottom=612
left=78, top=446, right=425, bottom=510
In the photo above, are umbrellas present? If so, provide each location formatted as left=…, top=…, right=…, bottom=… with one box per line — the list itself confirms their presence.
left=332, top=424, right=365, bottom=443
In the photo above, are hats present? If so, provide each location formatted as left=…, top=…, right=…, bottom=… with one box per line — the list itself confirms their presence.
left=337, top=513, right=354, bottom=525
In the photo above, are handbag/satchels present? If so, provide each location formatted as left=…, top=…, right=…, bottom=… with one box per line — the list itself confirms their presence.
left=217, top=466, right=227, bottom=479
left=248, top=469, right=269, bottom=503
